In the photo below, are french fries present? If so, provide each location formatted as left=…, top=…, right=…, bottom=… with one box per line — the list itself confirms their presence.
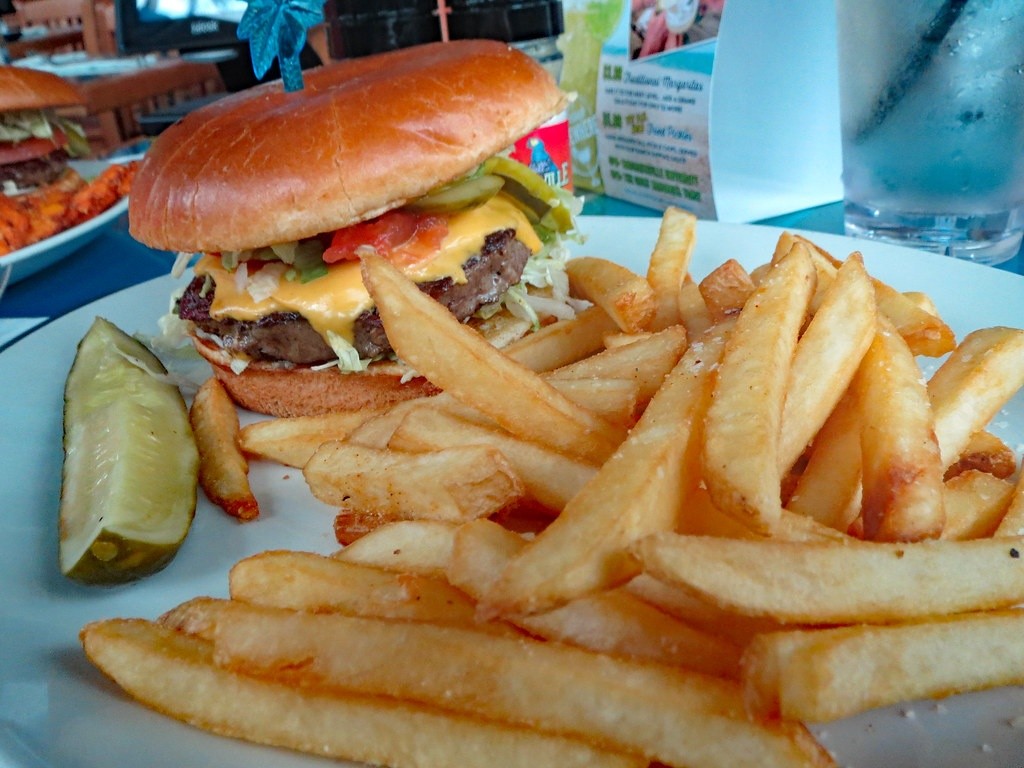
left=75, top=207, right=1024, bottom=768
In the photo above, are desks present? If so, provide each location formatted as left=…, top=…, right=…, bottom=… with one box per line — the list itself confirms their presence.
left=9, top=45, right=237, bottom=167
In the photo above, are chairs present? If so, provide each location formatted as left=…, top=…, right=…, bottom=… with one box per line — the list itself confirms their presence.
left=0, top=0, right=114, bottom=59
left=59, top=63, right=228, bottom=159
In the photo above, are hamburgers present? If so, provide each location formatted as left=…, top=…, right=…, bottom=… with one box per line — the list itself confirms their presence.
left=0, top=63, right=91, bottom=259
left=124, top=37, right=590, bottom=415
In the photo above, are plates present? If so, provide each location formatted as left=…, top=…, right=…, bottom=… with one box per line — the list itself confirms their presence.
left=0, top=161, right=129, bottom=285
left=0, top=218, right=1024, bottom=768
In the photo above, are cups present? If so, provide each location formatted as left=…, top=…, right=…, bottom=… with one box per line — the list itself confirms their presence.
left=835, top=0, right=1023, bottom=267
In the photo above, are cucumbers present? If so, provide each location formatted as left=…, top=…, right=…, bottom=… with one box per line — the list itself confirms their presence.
left=57, top=318, right=199, bottom=587
